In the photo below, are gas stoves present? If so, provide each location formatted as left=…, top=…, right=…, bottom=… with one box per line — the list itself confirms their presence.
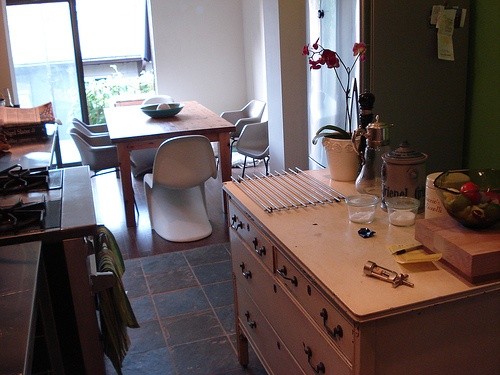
left=0, top=196, right=62, bottom=235
left=0, top=163, right=63, bottom=196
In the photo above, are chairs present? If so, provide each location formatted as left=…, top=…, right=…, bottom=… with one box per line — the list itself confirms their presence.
left=217, top=99, right=266, bottom=171
left=72, top=117, right=109, bottom=135
left=230, top=121, right=270, bottom=178
left=70, top=127, right=120, bottom=179
left=140, top=135, right=217, bottom=243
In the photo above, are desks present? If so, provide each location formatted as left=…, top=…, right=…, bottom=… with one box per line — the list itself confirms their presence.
left=0, top=122, right=62, bottom=173
left=102, top=101, right=236, bottom=226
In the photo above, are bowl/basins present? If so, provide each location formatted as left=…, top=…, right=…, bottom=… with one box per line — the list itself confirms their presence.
left=433, top=167, right=500, bottom=230
left=140, top=103, right=183, bottom=118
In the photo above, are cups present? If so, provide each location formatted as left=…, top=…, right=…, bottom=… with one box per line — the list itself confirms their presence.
left=385, top=196, right=421, bottom=227
left=345, top=193, right=379, bottom=223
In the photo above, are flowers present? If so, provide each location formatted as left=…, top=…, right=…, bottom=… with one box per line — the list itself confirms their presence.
left=302, top=38, right=368, bottom=145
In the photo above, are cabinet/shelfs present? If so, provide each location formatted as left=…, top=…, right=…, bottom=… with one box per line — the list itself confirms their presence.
left=221, top=167, right=500, bottom=375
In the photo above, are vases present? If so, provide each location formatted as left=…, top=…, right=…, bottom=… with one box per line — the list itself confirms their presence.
left=322, top=136, right=360, bottom=183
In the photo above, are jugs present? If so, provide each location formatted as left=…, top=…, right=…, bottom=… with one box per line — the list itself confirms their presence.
left=350, top=114, right=396, bottom=198
